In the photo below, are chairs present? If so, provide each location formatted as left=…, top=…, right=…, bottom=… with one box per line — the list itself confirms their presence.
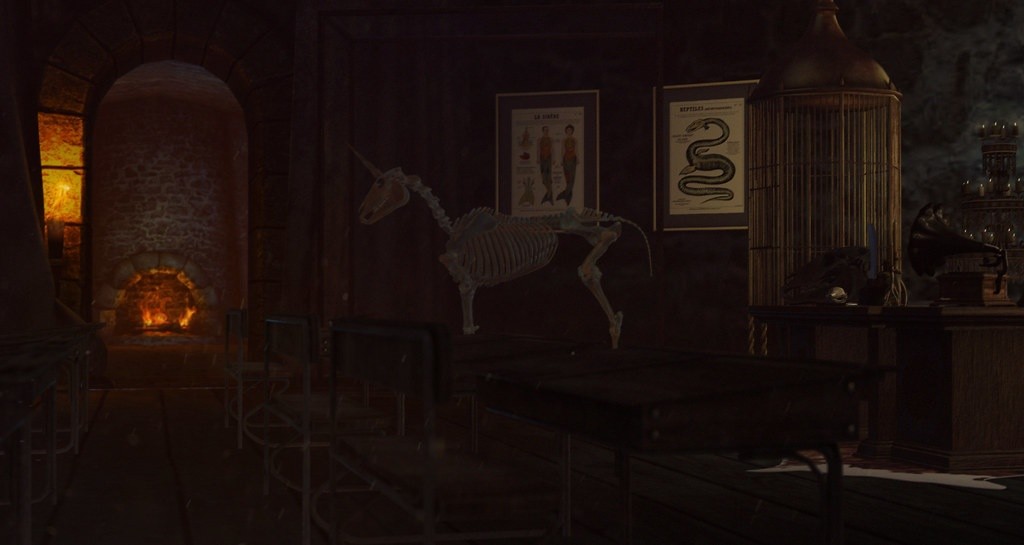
left=220, top=310, right=270, bottom=437
left=311, top=319, right=559, bottom=545
left=233, top=317, right=326, bottom=496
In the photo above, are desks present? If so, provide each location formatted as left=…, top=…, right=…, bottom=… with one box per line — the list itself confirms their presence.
left=473, top=347, right=910, bottom=544
left=0, top=320, right=115, bottom=543
left=754, top=303, right=1023, bottom=477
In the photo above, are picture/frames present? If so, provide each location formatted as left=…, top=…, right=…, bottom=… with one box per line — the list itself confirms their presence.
left=494, top=88, right=600, bottom=234
left=653, top=79, right=761, bottom=231
